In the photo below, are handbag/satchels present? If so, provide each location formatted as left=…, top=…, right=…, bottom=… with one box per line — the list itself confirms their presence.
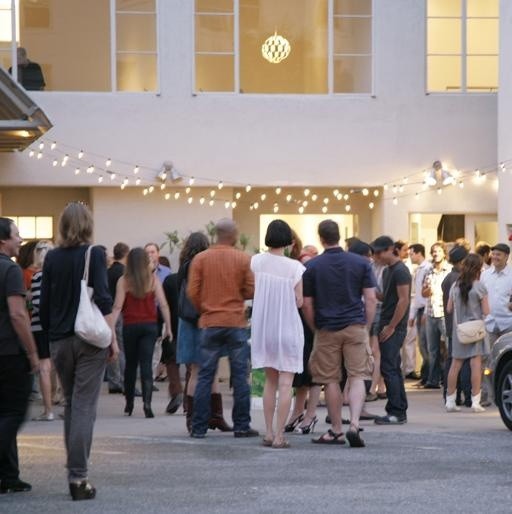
left=73, top=244, right=113, bottom=349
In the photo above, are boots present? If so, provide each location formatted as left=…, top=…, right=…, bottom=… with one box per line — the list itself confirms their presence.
left=208, top=391, right=235, bottom=433
left=184, top=393, right=198, bottom=435
left=470, top=389, right=485, bottom=414
left=141, top=369, right=154, bottom=419
left=123, top=362, right=138, bottom=416
left=444, top=390, right=462, bottom=414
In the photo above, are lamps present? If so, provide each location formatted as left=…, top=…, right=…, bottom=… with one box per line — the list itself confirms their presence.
left=426, top=162, right=453, bottom=188
left=262, top=2, right=291, bottom=65
left=156, top=160, right=183, bottom=183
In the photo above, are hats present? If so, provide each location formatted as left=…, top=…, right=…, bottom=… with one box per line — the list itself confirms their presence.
left=491, top=242, right=511, bottom=255
left=447, top=244, right=471, bottom=265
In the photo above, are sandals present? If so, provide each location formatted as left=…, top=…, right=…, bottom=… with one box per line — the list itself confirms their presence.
left=262, top=434, right=271, bottom=445
left=312, top=429, right=347, bottom=445
left=68, top=479, right=97, bottom=500
left=274, top=439, right=290, bottom=449
left=346, top=424, right=367, bottom=448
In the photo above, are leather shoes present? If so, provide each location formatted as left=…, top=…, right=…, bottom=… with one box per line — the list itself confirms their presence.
left=1, top=481, right=34, bottom=494
left=379, top=392, right=388, bottom=399
left=108, top=388, right=125, bottom=395
left=325, top=415, right=353, bottom=426
left=236, top=428, right=261, bottom=437
left=122, top=387, right=143, bottom=397
left=365, top=392, right=377, bottom=402
left=31, top=412, right=54, bottom=422
left=359, top=413, right=378, bottom=421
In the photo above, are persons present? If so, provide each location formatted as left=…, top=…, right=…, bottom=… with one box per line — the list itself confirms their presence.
left=474, top=242, right=493, bottom=274
left=394, top=243, right=419, bottom=379
left=285, top=228, right=321, bottom=433
left=481, top=244, right=512, bottom=407
left=162, top=272, right=183, bottom=413
left=344, top=236, right=362, bottom=252
left=8, top=48, right=44, bottom=91
left=112, top=247, right=171, bottom=417
left=144, top=244, right=172, bottom=285
left=89, top=246, right=124, bottom=395
left=1, top=216, right=40, bottom=492
left=370, top=236, right=411, bottom=423
left=16, top=240, right=37, bottom=289
left=107, top=242, right=128, bottom=349
left=326, top=242, right=379, bottom=426
left=40, top=201, right=118, bottom=500
left=407, top=244, right=432, bottom=386
left=250, top=219, right=304, bottom=447
left=175, top=231, right=232, bottom=433
left=421, top=240, right=452, bottom=389
left=445, top=255, right=488, bottom=413
left=303, top=221, right=377, bottom=444
left=188, top=219, right=259, bottom=437
left=443, top=243, right=474, bottom=407
left=31, top=243, right=55, bottom=422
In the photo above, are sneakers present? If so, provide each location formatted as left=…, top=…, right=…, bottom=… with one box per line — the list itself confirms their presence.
left=166, top=393, right=184, bottom=414
left=375, top=415, right=409, bottom=426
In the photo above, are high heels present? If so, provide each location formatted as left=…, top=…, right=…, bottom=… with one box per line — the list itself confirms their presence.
left=282, top=413, right=306, bottom=434
left=293, top=415, right=319, bottom=435
